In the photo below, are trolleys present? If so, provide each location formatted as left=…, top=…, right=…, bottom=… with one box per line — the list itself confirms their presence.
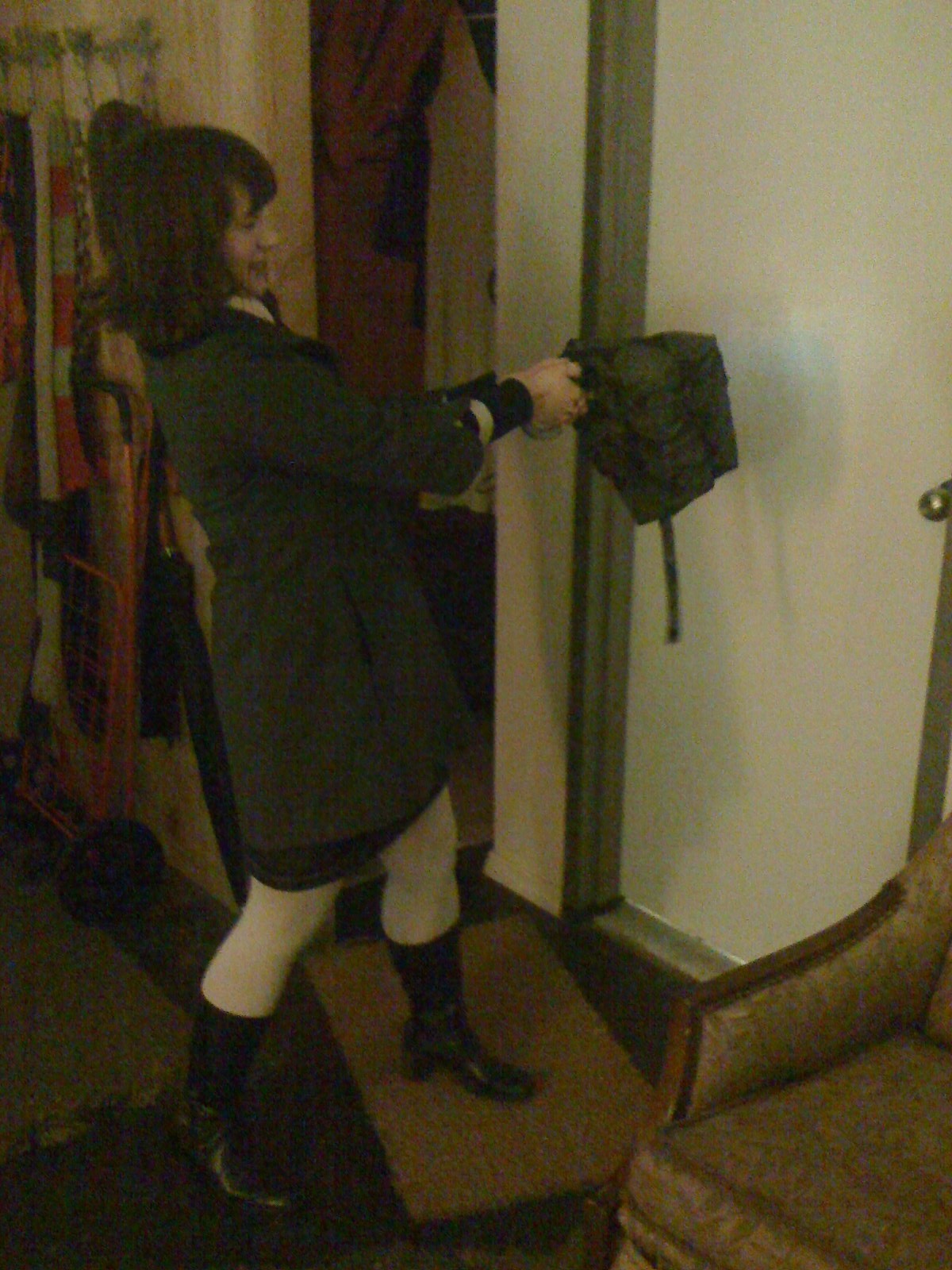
left=0, top=370, right=174, bottom=927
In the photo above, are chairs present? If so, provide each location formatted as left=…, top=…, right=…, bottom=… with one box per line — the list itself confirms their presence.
left=572, top=808, right=952, bottom=1270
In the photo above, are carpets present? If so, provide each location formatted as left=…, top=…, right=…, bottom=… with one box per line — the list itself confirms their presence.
left=304, top=910, right=653, bottom=1219
left=0, top=864, right=196, bottom=1161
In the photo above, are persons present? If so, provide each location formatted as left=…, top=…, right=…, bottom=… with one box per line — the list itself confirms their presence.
left=105, top=128, right=588, bottom=1213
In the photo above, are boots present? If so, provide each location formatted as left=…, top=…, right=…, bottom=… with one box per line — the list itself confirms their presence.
left=168, top=989, right=303, bottom=1219
left=382, top=914, right=540, bottom=1104
